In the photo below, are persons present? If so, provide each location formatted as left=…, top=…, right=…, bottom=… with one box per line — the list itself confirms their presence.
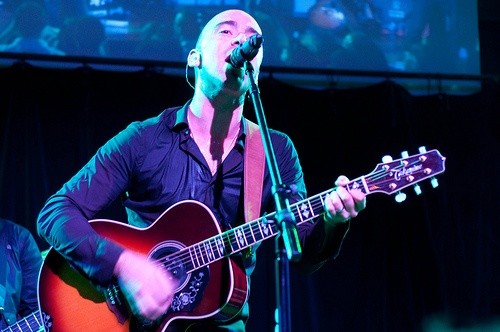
left=37, top=9, right=367, bottom=332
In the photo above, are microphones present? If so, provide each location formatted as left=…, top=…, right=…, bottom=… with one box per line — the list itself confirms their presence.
left=230, top=34, right=265, bottom=68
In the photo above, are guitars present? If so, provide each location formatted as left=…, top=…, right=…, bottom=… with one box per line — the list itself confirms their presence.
left=37, top=146, right=446, bottom=332
left=0, top=310, right=44, bottom=332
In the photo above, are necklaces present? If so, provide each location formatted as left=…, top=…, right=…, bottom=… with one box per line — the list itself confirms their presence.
left=187, top=107, right=240, bottom=173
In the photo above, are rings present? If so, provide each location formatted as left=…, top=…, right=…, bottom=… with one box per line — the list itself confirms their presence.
left=337, top=207, right=345, bottom=214
